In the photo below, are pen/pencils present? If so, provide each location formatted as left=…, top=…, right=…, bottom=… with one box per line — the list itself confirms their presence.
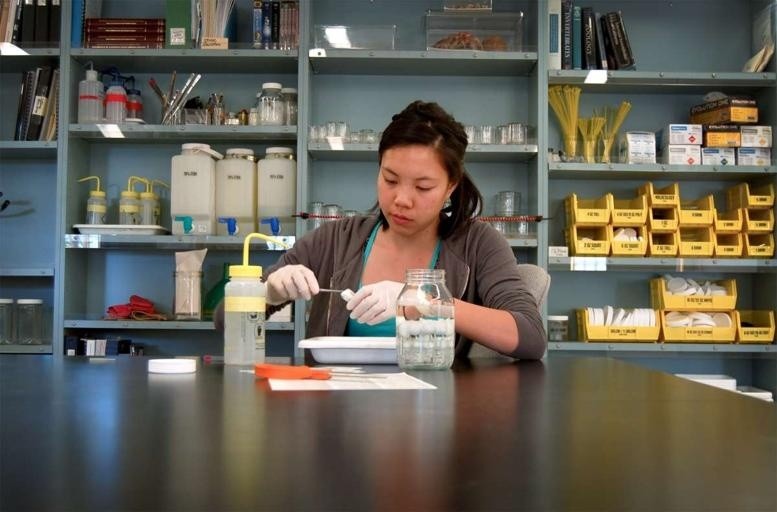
left=208, top=90, right=224, bottom=125
left=147, top=68, right=203, bottom=126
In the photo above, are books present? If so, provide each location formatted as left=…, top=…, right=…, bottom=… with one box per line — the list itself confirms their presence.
left=544, top=0, right=636, bottom=72
left=14, top=66, right=62, bottom=142
left=1, top=1, right=300, bottom=52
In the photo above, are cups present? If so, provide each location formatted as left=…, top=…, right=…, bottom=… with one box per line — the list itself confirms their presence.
left=302, top=119, right=383, bottom=144
left=173, top=270, right=204, bottom=319
left=309, top=201, right=359, bottom=228
left=467, top=121, right=534, bottom=145
left=489, top=188, right=529, bottom=236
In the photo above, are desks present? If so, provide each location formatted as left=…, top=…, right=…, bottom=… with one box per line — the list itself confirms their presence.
left=0, top=353, right=777, bottom=511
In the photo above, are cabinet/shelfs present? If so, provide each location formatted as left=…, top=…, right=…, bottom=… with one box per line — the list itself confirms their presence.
left=0, top=1, right=777, bottom=404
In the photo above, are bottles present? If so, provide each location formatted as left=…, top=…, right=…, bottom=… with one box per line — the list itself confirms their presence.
left=260, top=82, right=282, bottom=127
left=119, top=192, right=136, bottom=224
left=140, top=192, right=150, bottom=224
left=548, top=315, right=570, bottom=342
left=398, top=269, right=454, bottom=367
left=0, top=297, right=53, bottom=347
left=105, top=82, right=123, bottom=122
left=152, top=196, right=161, bottom=224
left=221, top=263, right=268, bottom=364
left=85, top=188, right=107, bottom=225
left=78, top=69, right=103, bottom=124
left=126, top=89, right=143, bottom=119
left=282, top=88, right=296, bottom=127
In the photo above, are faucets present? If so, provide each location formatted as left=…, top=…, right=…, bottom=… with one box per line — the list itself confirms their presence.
left=175, top=216, right=193, bottom=233
left=220, top=217, right=238, bottom=235
left=260, top=218, right=280, bottom=234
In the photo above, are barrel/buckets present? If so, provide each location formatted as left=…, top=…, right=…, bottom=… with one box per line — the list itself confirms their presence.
left=216, top=148, right=256, bottom=236
left=258, top=146, right=297, bottom=235
left=172, top=142, right=223, bottom=235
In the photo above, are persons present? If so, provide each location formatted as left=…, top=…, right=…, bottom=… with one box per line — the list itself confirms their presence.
left=213, top=101, right=547, bottom=362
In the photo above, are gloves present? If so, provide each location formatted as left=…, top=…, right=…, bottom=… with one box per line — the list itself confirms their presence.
left=262, top=264, right=319, bottom=308
left=344, top=272, right=407, bottom=326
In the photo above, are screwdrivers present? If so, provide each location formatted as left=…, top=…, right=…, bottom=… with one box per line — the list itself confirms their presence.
left=254, top=363, right=388, bottom=380
